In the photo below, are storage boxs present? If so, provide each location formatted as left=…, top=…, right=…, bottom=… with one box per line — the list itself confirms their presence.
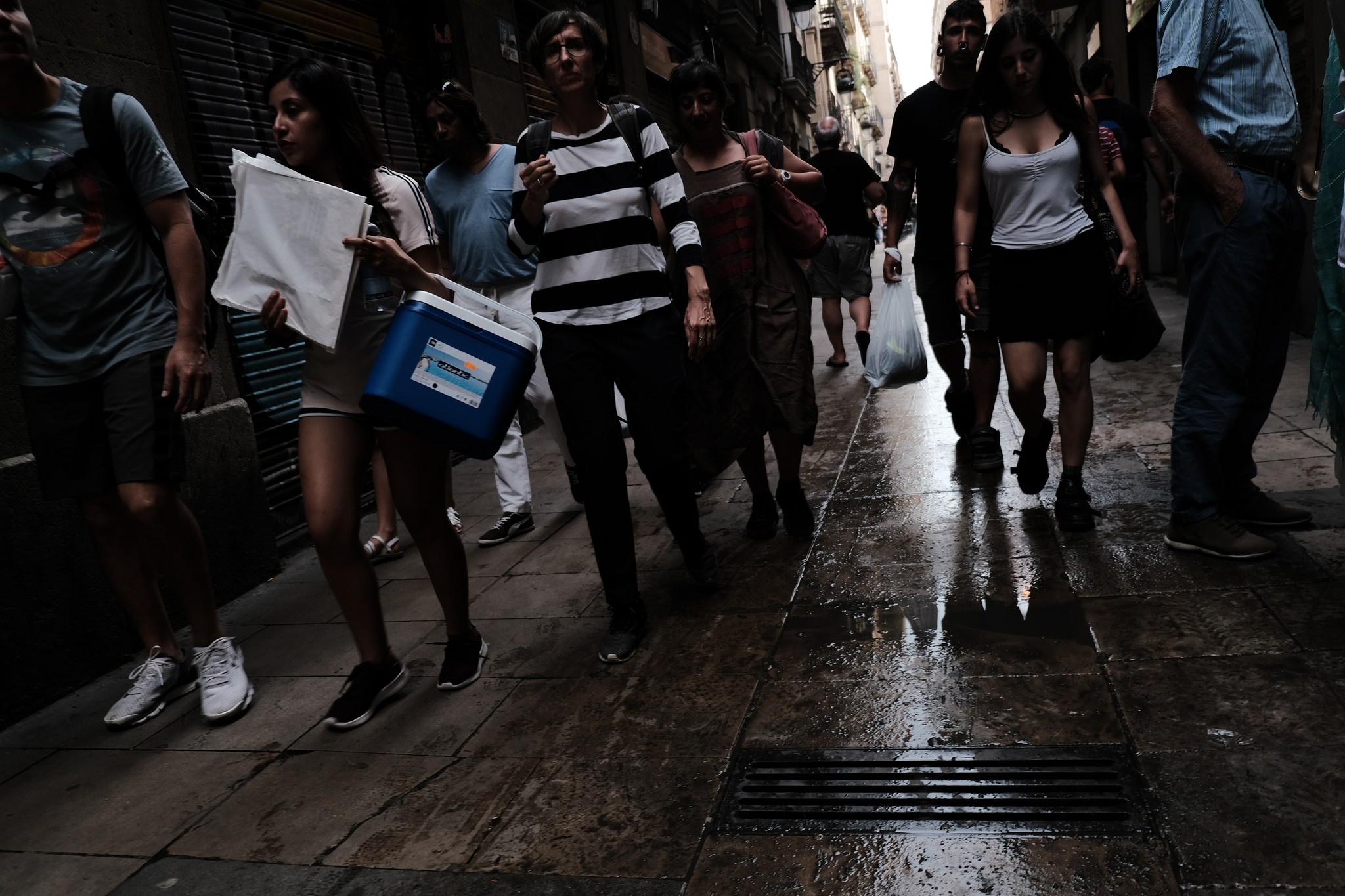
left=358, top=272, right=543, bottom=461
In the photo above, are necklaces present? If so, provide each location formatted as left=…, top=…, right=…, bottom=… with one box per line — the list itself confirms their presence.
left=1003, top=106, right=1047, bottom=120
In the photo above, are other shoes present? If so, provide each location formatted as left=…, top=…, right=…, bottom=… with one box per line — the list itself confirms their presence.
left=825, top=355, right=849, bottom=367
left=1009, top=417, right=1053, bottom=494
left=746, top=476, right=814, bottom=541
left=1055, top=474, right=1102, bottom=529
left=856, top=329, right=871, bottom=367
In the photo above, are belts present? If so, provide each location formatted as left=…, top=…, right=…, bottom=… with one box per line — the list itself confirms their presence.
left=1214, top=147, right=1297, bottom=185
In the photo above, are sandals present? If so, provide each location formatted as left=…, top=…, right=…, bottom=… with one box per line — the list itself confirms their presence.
left=446, top=506, right=464, bottom=533
left=362, top=534, right=405, bottom=565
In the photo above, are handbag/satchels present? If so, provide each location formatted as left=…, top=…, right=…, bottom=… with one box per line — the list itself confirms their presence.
left=735, top=131, right=828, bottom=260
left=861, top=247, right=928, bottom=389
left=1083, top=187, right=1166, bottom=360
left=1295, top=84, right=1325, bottom=201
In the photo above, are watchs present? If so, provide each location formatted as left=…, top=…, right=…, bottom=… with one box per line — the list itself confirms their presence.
left=778, top=170, right=791, bottom=187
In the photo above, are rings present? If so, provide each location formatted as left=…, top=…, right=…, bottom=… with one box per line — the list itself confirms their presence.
left=536, top=178, right=544, bottom=186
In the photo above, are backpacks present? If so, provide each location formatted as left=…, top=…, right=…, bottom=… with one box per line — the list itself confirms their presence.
left=83, top=80, right=223, bottom=355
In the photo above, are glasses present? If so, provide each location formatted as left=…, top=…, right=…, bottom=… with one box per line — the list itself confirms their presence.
left=536, top=38, right=589, bottom=66
left=434, top=82, right=459, bottom=94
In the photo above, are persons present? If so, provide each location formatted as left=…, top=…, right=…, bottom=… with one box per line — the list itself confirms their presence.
left=363, top=437, right=461, bottom=565
left=0, top=0, right=259, bottom=731
left=412, top=76, right=584, bottom=544
left=506, top=8, right=715, bottom=663
left=256, top=54, right=489, bottom=728
left=606, top=0, right=1345, bottom=560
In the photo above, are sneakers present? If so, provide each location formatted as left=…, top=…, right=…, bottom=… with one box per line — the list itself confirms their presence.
left=664, top=514, right=717, bottom=581
left=1226, top=490, right=1313, bottom=526
left=1165, top=513, right=1276, bottom=559
left=103, top=645, right=201, bottom=728
left=563, top=459, right=585, bottom=504
left=435, top=627, right=488, bottom=692
left=598, top=623, right=649, bottom=664
left=324, top=644, right=409, bottom=729
left=477, top=512, right=535, bottom=544
left=945, top=368, right=977, bottom=437
left=190, top=634, right=255, bottom=726
left=967, top=424, right=1003, bottom=470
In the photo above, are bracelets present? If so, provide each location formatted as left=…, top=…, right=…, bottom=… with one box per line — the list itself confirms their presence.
left=953, top=269, right=971, bottom=284
left=954, top=241, right=973, bottom=252
